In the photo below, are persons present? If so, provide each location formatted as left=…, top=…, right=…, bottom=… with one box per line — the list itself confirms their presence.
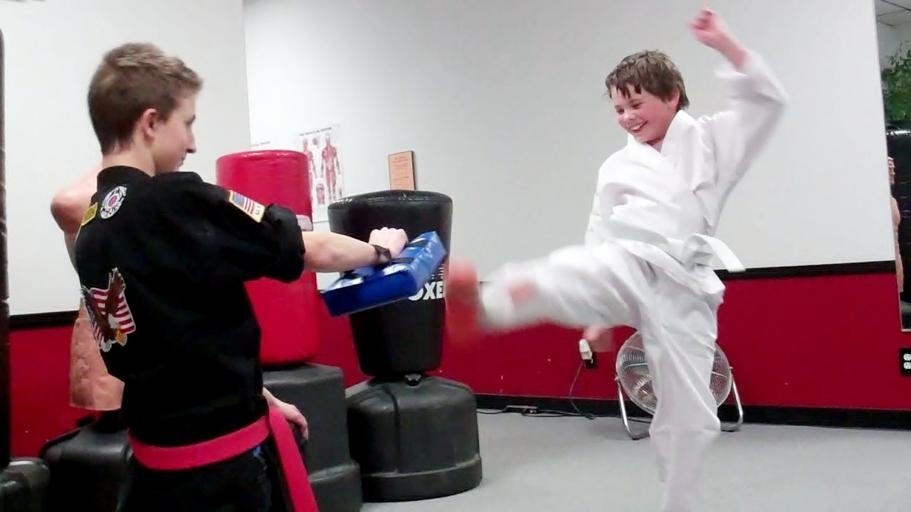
left=48, top=165, right=126, bottom=412
left=887, top=157, right=911, bottom=315
left=73, top=43, right=410, bottom=512
left=445, top=6, right=788, bottom=512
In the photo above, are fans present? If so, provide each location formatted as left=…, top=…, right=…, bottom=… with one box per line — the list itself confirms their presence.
left=614, top=329, right=744, bottom=441
